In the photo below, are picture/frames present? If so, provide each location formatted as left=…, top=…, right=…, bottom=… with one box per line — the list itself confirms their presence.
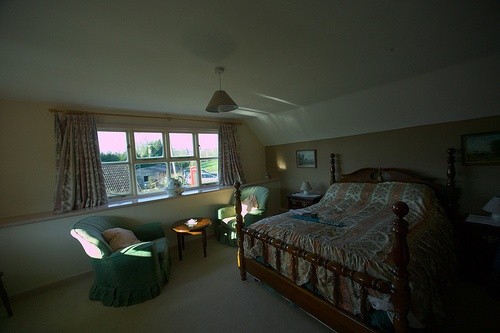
left=460, top=130, right=500, bottom=167
left=296, top=149, right=317, bottom=168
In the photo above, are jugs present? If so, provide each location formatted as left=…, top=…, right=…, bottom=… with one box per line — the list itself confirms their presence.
left=168, top=176, right=183, bottom=188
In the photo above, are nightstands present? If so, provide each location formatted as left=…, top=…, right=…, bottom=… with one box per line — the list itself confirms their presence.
left=287, top=193, right=324, bottom=211
left=461, top=213, right=500, bottom=273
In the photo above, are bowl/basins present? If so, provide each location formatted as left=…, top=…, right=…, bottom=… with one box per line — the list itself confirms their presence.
left=165, top=188, right=184, bottom=196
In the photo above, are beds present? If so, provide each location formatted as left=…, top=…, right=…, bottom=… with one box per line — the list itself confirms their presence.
left=232, top=148, right=460, bottom=333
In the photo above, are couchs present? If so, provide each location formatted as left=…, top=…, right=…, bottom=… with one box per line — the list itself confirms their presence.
left=70, top=215, right=172, bottom=307
left=216, top=185, right=269, bottom=248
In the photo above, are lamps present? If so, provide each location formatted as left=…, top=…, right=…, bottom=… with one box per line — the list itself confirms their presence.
left=482, top=196, right=500, bottom=222
left=300, top=181, right=311, bottom=194
left=206, top=67, right=238, bottom=114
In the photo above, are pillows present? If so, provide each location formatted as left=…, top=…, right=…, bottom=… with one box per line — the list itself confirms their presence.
left=101, top=228, right=141, bottom=251
left=241, top=195, right=258, bottom=218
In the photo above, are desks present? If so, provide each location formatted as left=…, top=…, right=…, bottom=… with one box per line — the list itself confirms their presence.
left=172, top=217, right=212, bottom=261
